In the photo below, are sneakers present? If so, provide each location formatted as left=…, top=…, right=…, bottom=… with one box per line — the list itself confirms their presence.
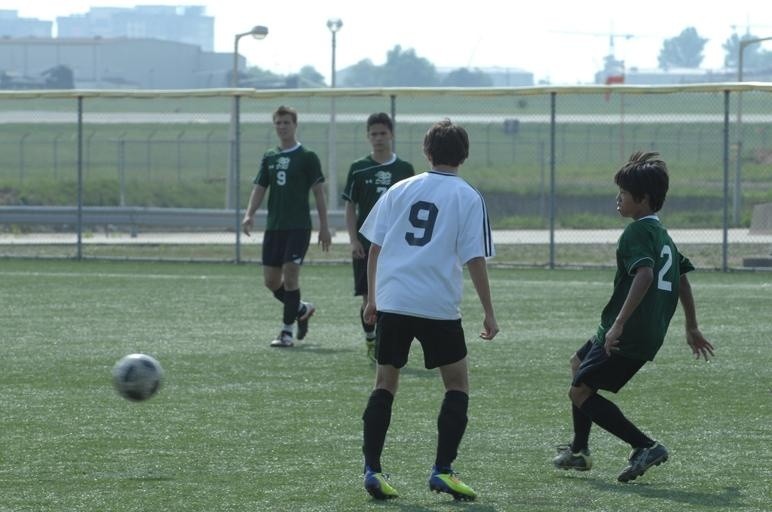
left=366, top=339, right=376, bottom=362
left=552, top=446, right=593, bottom=471
left=270, top=331, right=293, bottom=347
left=430, top=465, right=477, bottom=501
left=296, top=302, right=315, bottom=340
left=617, top=440, right=668, bottom=482
left=363, top=464, right=399, bottom=498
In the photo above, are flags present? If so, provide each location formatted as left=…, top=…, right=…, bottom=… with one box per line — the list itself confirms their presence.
left=604, top=76, right=624, bottom=103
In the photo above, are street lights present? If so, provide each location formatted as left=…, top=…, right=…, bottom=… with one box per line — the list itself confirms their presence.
left=233, top=26, right=270, bottom=264
left=323, top=14, right=351, bottom=214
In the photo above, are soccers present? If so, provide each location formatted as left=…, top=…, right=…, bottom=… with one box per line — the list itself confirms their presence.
left=112, top=354, right=162, bottom=401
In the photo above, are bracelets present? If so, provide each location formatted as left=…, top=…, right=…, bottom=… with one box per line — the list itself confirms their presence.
left=615, top=315, right=626, bottom=328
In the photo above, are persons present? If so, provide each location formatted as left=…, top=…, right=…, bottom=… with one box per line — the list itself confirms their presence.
left=341, top=111, right=415, bottom=364
left=358, top=117, right=499, bottom=500
left=552, top=149, right=715, bottom=483
left=241, top=104, right=331, bottom=347
